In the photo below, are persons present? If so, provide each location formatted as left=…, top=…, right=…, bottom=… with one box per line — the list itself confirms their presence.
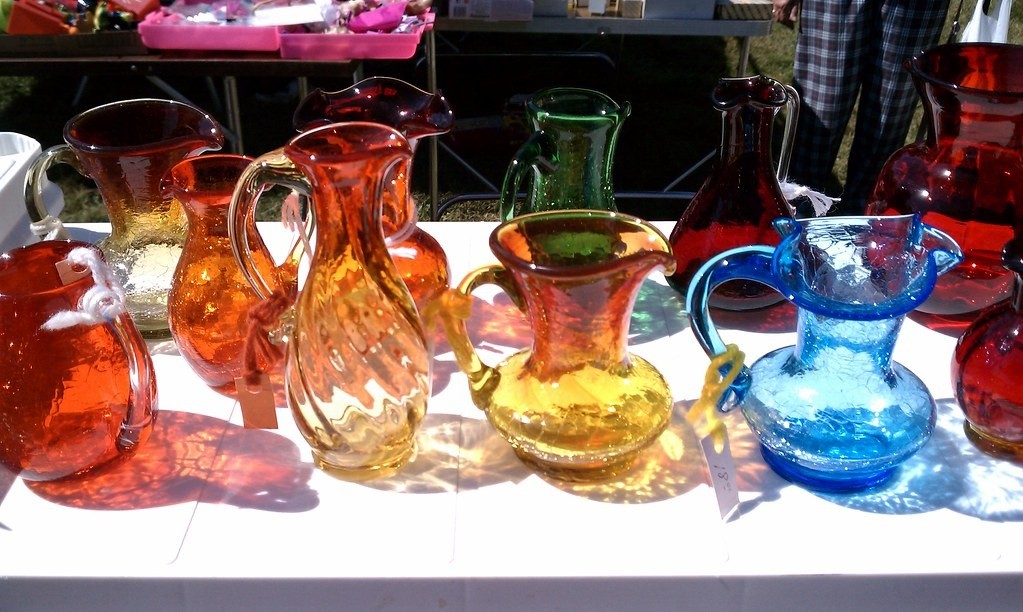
left=773, top=0, right=952, bottom=218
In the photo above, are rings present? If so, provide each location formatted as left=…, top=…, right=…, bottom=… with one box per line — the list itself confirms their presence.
left=772, top=10, right=776, bottom=13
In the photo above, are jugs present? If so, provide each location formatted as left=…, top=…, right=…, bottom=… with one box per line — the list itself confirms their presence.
left=24, top=42, right=1023, bottom=496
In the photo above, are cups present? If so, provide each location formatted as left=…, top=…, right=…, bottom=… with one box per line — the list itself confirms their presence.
left=0, top=240, right=157, bottom=482
